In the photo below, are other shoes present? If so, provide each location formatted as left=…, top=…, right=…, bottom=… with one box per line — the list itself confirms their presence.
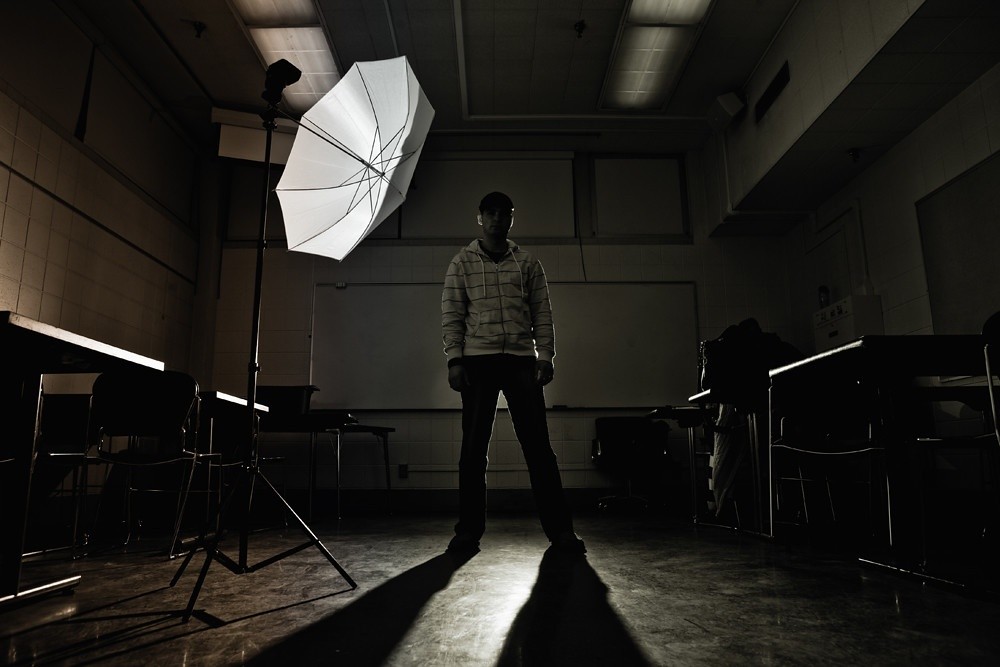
left=554, top=528, right=584, bottom=550
left=446, top=535, right=480, bottom=554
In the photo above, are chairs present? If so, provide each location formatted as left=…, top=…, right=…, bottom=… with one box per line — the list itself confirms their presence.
left=592, top=311, right=1000, bottom=596
left=20, top=371, right=288, bottom=559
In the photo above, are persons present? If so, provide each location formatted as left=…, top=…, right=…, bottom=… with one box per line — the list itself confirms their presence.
left=442, top=191, right=587, bottom=556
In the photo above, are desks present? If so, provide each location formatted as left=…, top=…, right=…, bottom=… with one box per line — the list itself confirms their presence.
left=257, top=427, right=397, bottom=521
left=641, top=401, right=716, bottom=525
left=768, top=330, right=983, bottom=588
left=177, top=392, right=271, bottom=546
left=0, top=311, right=166, bottom=607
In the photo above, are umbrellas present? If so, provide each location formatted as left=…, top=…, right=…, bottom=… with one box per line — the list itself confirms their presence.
left=269, top=55, right=435, bottom=262
